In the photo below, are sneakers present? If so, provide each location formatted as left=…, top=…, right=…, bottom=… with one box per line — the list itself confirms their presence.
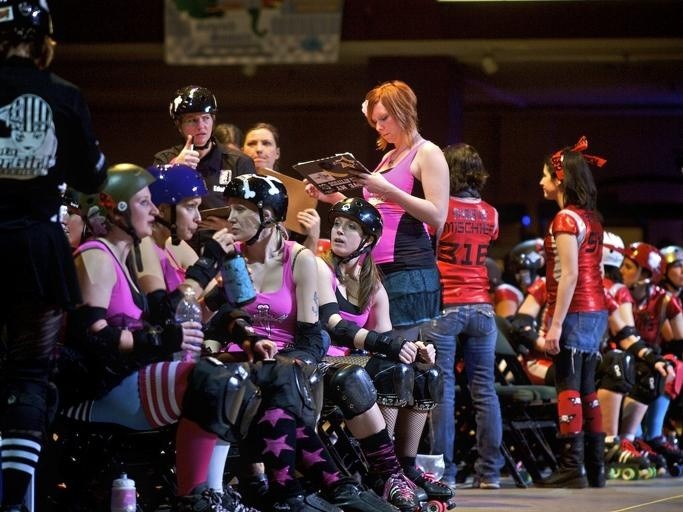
left=437, top=476, right=458, bottom=492
left=476, top=476, right=502, bottom=491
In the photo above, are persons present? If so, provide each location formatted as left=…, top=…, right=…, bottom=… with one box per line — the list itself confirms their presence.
left=36, top=82, right=682, bottom=512
left=0, top=0, right=110, bottom=512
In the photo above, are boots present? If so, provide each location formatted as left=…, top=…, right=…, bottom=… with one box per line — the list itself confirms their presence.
left=580, top=430, right=607, bottom=489
left=541, top=430, right=589, bottom=489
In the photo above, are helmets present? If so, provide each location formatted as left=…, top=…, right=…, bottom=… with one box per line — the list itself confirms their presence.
left=167, top=85, right=220, bottom=118
left=598, top=232, right=627, bottom=269
left=327, top=195, right=384, bottom=237
left=62, top=184, right=110, bottom=229
left=142, top=163, right=209, bottom=209
left=616, top=241, right=666, bottom=284
left=659, top=245, right=683, bottom=270
left=0, top=1, right=63, bottom=42
left=508, top=239, right=547, bottom=272
left=220, top=173, right=290, bottom=222
left=481, top=259, right=503, bottom=286
left=97, top=161, right=156, bottom=214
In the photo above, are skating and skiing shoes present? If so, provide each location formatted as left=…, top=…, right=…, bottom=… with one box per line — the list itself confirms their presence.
left=404, top=465, right=464, bottom=511
left=237, top=474, right=291, bottom=511
left=370, top=465, right=421, bottom=512
left=648, top=438, right=681, bottom=477
left=600, top=438, right=652, bottom=485
left=330, top=485, right=401, bottom=512
left=283, top=491, right=344, bottom=512
left=181, top=486, right=229, bottom=512
left=632, top=437, right=668, bottom=480
left=217, top=485, right=260, bottom=512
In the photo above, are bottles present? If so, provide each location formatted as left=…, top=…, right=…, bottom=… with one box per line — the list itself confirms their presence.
left=110, top=473, right=138, bottom=512
left=175, top=285, right=203, bottom=363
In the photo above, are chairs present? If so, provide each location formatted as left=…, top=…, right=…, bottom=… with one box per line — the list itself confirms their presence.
left=496, top=326, right=559, bottom=484
left=453, top=318, right=537, bottom=489
